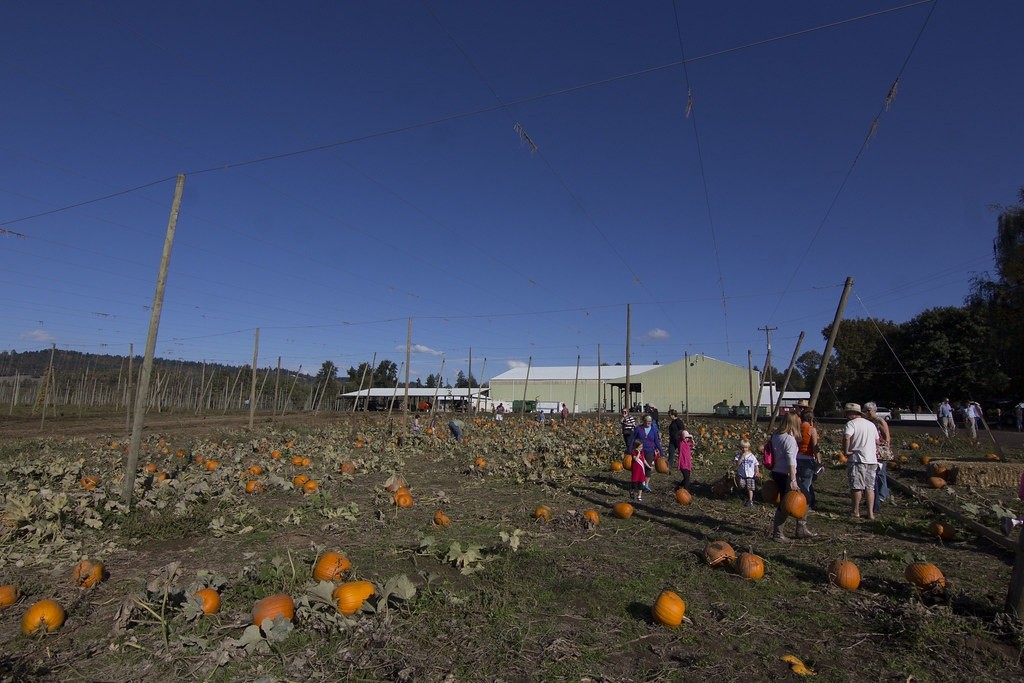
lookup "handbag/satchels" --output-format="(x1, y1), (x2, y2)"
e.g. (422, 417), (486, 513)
(763, 439), (773, 469)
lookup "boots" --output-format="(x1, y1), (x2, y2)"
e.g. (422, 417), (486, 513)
(796, 513), (818, 538)
(773, 510), (792, 544)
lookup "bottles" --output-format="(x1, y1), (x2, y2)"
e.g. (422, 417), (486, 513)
(815, 466), (824, 475)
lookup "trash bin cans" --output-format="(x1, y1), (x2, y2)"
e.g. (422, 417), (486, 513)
(733, 406), (749, 417)
(715, 406), (729, 415)
(513, 400), (538, 413)
(753, 406), (767, 417)
(780, 407), (794, 415)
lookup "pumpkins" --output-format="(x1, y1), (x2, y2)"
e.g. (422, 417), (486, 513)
(0, 417), (1001, 636)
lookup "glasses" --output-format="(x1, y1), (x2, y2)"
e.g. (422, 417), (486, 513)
(864, 409), (872, 413)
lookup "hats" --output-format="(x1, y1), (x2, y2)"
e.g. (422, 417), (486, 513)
(840, 402), (868, 417)
(792, 399), (812, 411)
(677, 429), (692, 439)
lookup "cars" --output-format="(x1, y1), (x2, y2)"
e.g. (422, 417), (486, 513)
(357, 398), (404, 412)
(876, 406), (893, 421)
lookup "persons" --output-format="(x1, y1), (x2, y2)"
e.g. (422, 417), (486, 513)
(994, 408), (1002, 431)
(535, 411), (545, 421)
(862, 402), (894, 513)
(770, 412), (818, 543)
(413, 415), (420, 434)
(620, 407), (636, 445)
(734, 439), (759, 506)
(629, 439), (654, 501)
(676, 430), (695, 489)
(496, 403), (504, 423)
(550, 409), (554, 419)
(561, 404), (569, 424)
(842, 403), (880, 519)
(796, 409), (821, 504)
(648, 403), (658, 424)
(628, 414), (664, 491)
(939, 398), (956, 435)
(447, 419), (461, 441)
(963, 400), (982, 438)
(667, 409), (685, 469)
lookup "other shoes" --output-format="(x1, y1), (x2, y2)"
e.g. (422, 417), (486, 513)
(807, 509), (816, 513)
(1001, 516), (1013, 536)
(851, 512), (860, 518)
(629, 489), (635, 499)
(642, 480), (652, 492)
(637, 495), (642, 501)
(745, 500), (753, 507)
(868, 513), (875, 519)
